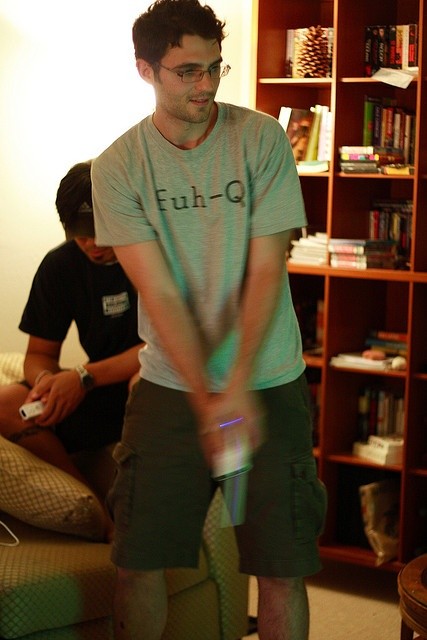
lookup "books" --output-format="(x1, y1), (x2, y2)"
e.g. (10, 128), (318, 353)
(347, 387), (403, 462)
(328, 201), (415, 269)
(365, 23), (419, 81)
(278, 104), (331, 174)
(284, 30), (293, 78)
(331, 331), (408, 372)
(286, 231), (327, 267)
(315, 300), (325, 357)
(335, 94), (414, 173)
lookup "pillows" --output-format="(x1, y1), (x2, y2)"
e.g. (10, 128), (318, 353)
(0, 436), (111, 541)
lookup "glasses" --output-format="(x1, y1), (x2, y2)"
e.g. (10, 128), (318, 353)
(149, 57), (232, 84)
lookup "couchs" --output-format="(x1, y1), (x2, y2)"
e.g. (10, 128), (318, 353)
(0, 351), (247, 640)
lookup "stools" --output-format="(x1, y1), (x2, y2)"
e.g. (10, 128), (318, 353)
(397, 556), (427, 640)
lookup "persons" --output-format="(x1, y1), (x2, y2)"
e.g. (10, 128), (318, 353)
(91, 0), (327, 639)
(0, 160), (146, 504)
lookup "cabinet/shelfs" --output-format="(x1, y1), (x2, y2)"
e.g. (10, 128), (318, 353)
(254, 1), (427, 604)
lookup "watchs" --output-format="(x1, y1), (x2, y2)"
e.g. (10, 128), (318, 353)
(75, 365), (96, 391)
